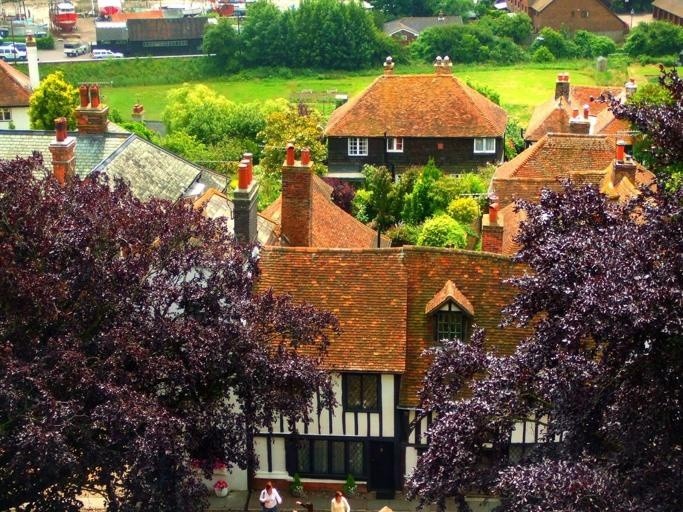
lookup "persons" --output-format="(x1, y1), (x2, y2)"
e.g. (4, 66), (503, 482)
(258, 481), (284, 512)
(330, 489), (350, 512)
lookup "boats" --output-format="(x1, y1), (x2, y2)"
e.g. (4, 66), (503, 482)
(48, 1), (77, 33)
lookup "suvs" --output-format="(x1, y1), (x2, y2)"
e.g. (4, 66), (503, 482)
(0, 42), (26, 61)
(63, 41), (85, 57)
(92, 49), (123, 59)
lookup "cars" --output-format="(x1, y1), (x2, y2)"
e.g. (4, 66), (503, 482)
(95, 16), (111, 23)
